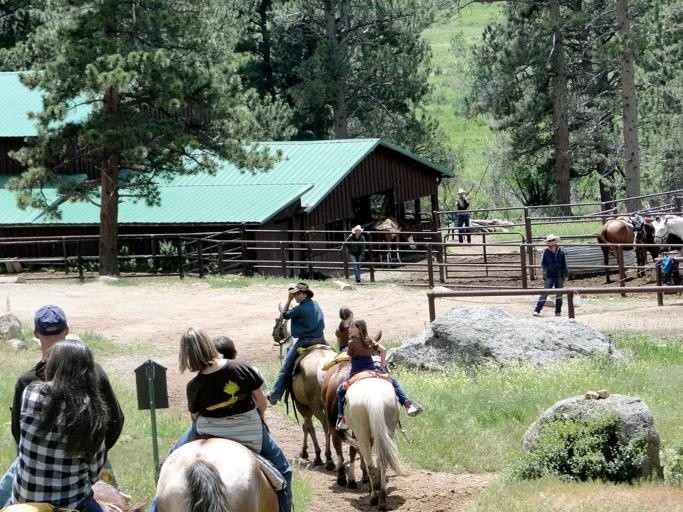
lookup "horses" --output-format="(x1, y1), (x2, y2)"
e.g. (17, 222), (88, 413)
(652, 213), (683, 247)
(154, 436), (281, 512)
(271, 300), (340, 472)
(341, 351), (410, 512)
(596, 216), (662, 284)
(361, 215), (417, 269)
(319, 327), (383, 489)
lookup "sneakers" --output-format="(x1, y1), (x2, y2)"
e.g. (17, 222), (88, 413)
(335, 420), (349, 430)
(406, 407), (423, 416)
(265, 391), (277, 405)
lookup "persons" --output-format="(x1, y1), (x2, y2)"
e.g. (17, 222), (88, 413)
(629, 216), (648, 278)
(375, 216), (403, 258)
(531, 232), (567, 319)
(650, 215), (668, 253)
(343, 225), (367, 283)
(266, 278), (329, 407)
(454, 188), (471, 244)
(8, 303), (124, 512)
(158, 324), (295, 509)
(656, 251), (675, 285)
(331, 319), (424, 434)
(210, 335), (239, 360)
(337, 303), (383, 352)
(0, 339), (109, 512)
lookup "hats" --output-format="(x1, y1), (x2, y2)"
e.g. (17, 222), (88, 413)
(543, 234), (560, 242)
(351, 224), (364, 234)
(33, 304), (66, 336)
(456, 188), (467, 194)
(287, 283), (313, 298)
